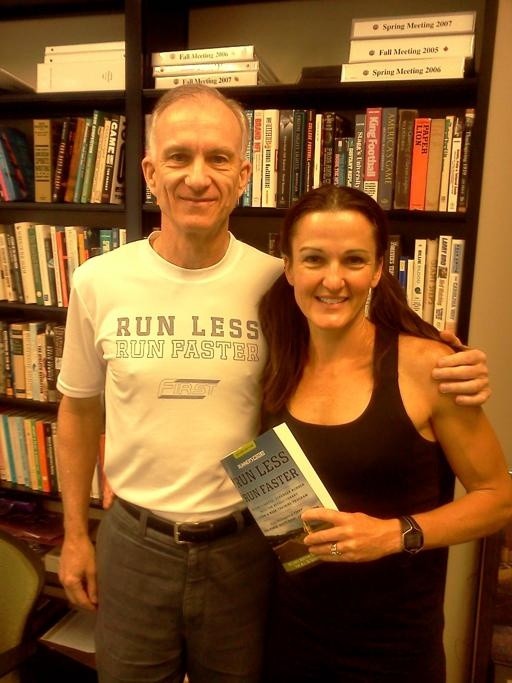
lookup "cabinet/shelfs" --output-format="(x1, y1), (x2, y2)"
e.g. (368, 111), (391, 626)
(0, 0), (499, 683)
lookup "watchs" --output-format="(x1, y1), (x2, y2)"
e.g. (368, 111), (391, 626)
(400, 513), (424, 554)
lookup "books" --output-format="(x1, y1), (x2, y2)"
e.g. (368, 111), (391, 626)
(238, 108), (483, 212)
(1, 222), (126, 307)
(1, 321), (65, 403)
(39, 611), (97, 668)
(219, 421), (339, 576)
(2, 522), (95, 598)
(341, 10), (485, 83)
(1, 111), (128, 205)
(152, 46), (282, 89)
(36, 41), (126, 92)
(1, 407), (107, 498)
(267, 231), (474, 340)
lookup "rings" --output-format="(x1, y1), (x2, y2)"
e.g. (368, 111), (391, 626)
(331, 543), (342, 557)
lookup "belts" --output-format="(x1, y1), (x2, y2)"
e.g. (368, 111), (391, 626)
(119, 499), (254, 542)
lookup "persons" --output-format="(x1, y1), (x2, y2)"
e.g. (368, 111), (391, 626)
(54, 86), (492, 677)
(267, 185), (512, 677)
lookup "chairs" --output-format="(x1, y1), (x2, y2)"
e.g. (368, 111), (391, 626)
(0, 528), (47, 683)
